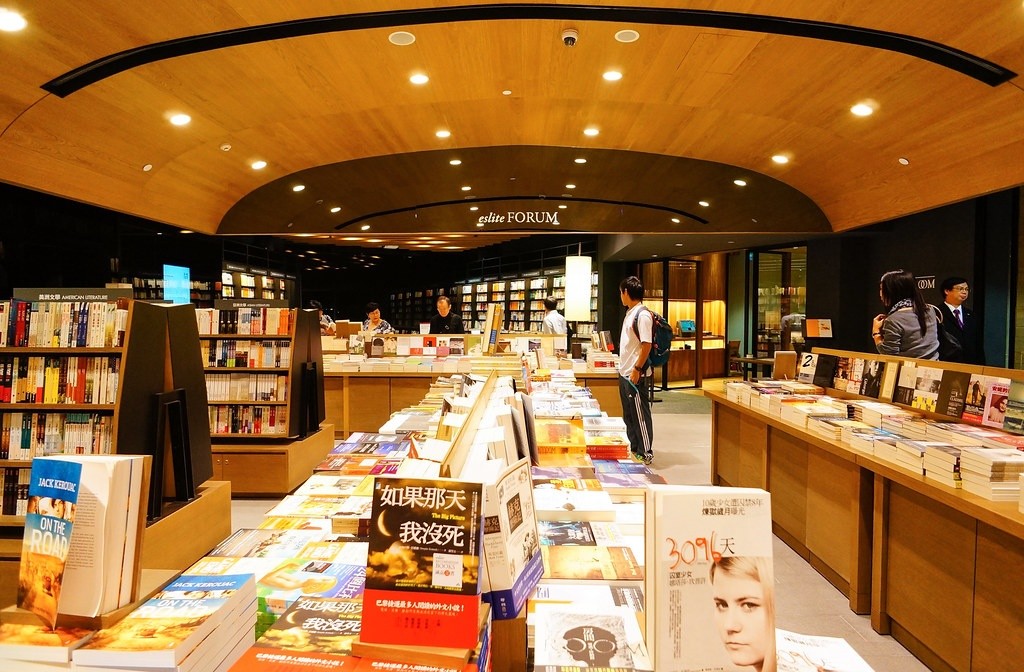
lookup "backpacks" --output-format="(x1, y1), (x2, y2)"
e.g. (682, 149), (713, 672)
(632, 306), (673, 372)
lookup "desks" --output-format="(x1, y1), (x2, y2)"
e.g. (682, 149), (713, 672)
(855, 366), (1024, 672)
(732, 358), (774, 381)
(703, 346), (985, 614)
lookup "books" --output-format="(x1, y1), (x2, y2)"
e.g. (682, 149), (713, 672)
(0, 272), (1024, 672)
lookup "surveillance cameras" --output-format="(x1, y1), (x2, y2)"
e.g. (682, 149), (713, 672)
(562, 28), (579, 48)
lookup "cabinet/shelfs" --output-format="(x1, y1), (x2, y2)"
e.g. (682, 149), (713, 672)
(390, 271), (599, 339)
(0, 269), (334, 570)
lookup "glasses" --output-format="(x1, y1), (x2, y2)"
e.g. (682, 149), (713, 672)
(947, 287), (970, 292)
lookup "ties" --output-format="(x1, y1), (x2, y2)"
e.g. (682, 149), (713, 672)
(953, 309), (963, 331)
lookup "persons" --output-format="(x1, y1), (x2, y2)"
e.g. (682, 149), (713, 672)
(541, 296), (567, 334)
(972, 381), (981, 404)
(560, 308), (574, 353)
(872, 269), (940, 361)
(781, 303), (806, 338)
(935, 277), (986, 366)
(26, 496), (41, 514)
(709, 556), (777, 672)
(363, 302), (391, 334)
(51, 498), (66, 519)
(980, 393), (986, 407)
(430, 296), (466, 334)
(67, 503), (76, 523)
(859, 360), (885, 399)
(618, 276), (655, 465)
(311, 302), (336, 336)
(988, 396), (1008, 423)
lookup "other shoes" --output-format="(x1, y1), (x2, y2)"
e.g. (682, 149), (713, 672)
(633, 452), (653, 465)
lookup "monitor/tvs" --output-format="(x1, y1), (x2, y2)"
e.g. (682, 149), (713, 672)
(677, 319), (696, 337)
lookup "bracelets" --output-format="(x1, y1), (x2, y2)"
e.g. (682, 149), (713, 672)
(872, 332), (881, 338)
(635, 366), (642, 371)
